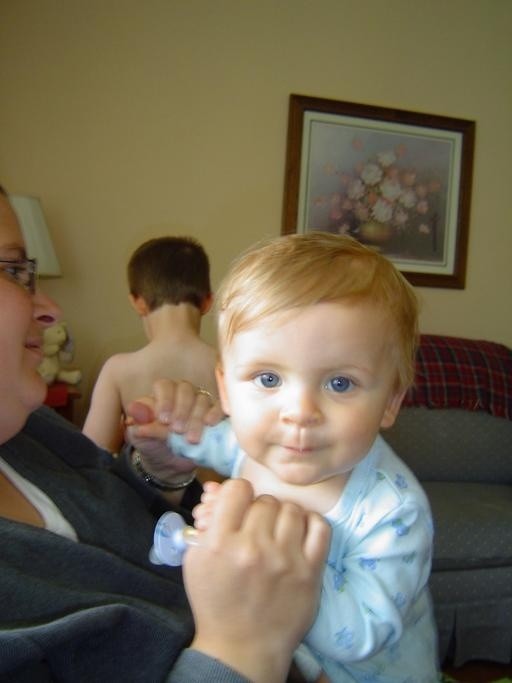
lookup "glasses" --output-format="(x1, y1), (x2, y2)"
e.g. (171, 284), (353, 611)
(0, 255), (37, 296)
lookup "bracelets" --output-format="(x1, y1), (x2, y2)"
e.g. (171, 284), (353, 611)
(135, 449), (197, 492)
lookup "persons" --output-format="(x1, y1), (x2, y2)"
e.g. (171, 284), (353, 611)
(82, 237), (220, 455)
(124, 232), (437, 683)
(0, 182), (333, 683)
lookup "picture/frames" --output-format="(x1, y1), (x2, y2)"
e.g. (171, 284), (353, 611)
(280, 94), (477, 292)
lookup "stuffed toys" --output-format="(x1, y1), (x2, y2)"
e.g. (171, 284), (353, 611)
(37, 324), (81, 385)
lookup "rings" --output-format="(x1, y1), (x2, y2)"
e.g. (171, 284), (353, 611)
(195, 387), (215, 407)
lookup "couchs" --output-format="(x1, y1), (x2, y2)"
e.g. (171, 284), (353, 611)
(378, 335), (510, 668)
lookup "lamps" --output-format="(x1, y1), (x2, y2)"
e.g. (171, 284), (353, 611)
(11, 196), (64, 279)
